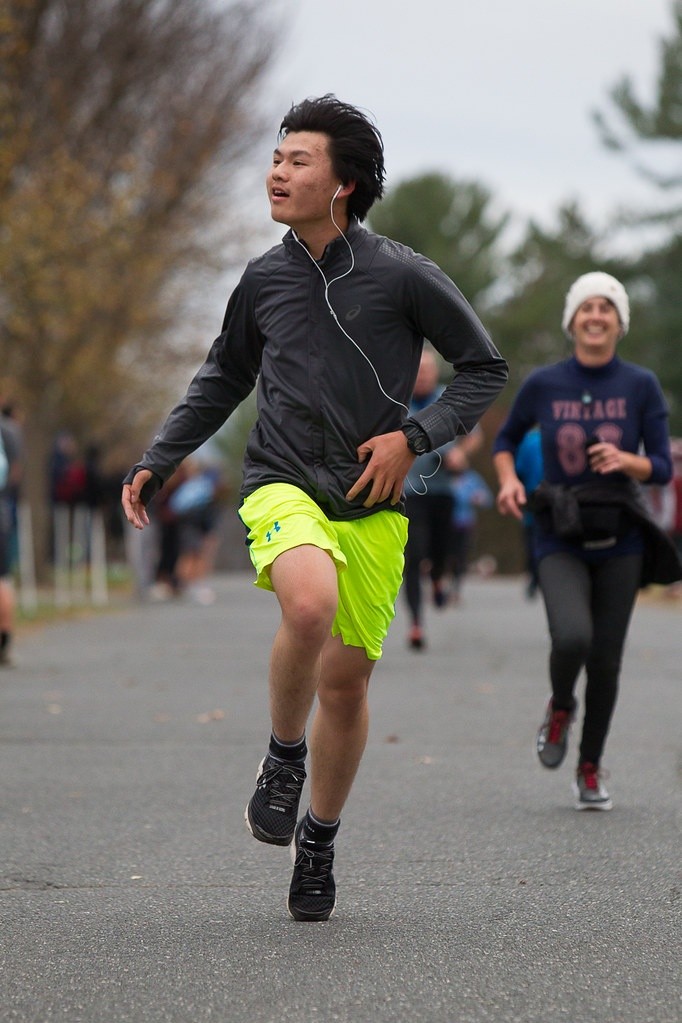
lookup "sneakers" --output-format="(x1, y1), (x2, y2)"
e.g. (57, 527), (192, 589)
(535, 697), (577, 769)
(287, 820), (335, 924)
(245, 754), (307, 845)
(570, 769), (616, 811)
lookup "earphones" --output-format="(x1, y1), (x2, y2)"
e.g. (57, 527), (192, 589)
(334, 184), (345, 199)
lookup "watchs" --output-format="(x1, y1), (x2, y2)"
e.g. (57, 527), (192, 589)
(401, 422), (429, 456)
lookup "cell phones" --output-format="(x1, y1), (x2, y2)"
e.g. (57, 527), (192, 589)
(584, 435), (606, 463)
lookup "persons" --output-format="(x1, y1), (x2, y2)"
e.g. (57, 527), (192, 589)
(445, 446), (491, 603)
(493, 271), (675, 814)
(404, 356), (456, 653)
(0, 389), (29, 667)
(42, 405), (233, 605)
(125, 91), (509, 921)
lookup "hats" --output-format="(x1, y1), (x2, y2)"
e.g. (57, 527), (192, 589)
(562, 271), (633, 340)
